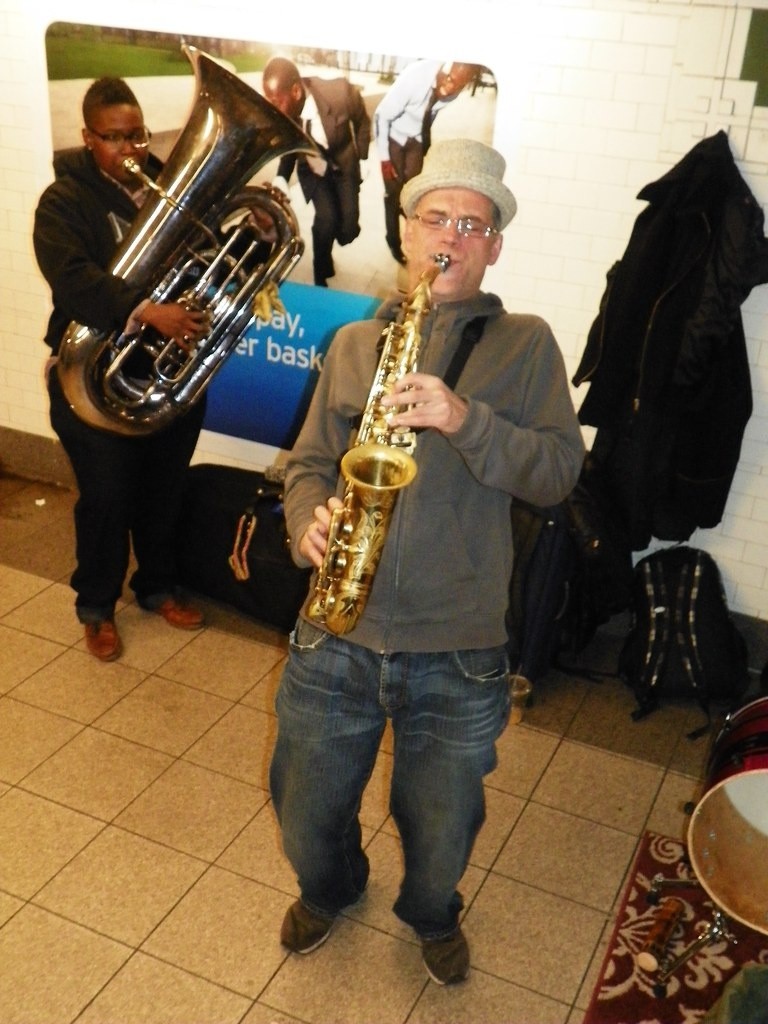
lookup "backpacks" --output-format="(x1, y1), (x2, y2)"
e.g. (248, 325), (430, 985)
(618, 546), (752, 740)
(505, 451), (632, 709)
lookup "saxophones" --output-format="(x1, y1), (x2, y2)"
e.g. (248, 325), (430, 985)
(300, 251), (451, 636)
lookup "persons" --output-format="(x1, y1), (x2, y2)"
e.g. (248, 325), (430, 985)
(374, 61), (483, 266)
(31, 73), (289, 660)
(268, 136), (585, 987)
(261, 57), (373, 286)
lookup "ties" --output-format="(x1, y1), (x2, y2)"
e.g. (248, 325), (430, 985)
(422, 95), (439, 156)
(306, 119), (333, 169)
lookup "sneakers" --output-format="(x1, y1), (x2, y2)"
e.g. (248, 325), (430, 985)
(280, 899), (335, 954)
(421, 927), (470, 985)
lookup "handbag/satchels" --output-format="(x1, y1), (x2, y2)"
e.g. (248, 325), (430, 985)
(178, 464), (315, 631)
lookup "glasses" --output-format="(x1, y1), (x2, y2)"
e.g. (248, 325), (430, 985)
(86, 125), (151, 150)
(412, 214), (497, 238)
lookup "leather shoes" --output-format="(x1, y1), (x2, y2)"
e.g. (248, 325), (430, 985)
(392, 245), (407, 264)
(85, 617), (122, 662)
(154, 593), (204, 629)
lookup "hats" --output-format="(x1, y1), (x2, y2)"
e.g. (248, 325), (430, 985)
(400, 139), (517, 233)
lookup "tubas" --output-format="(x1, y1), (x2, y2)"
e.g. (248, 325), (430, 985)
(53, 38), (334, 438)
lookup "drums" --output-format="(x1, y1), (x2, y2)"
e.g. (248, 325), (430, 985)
(683, 695), (768, 939)
